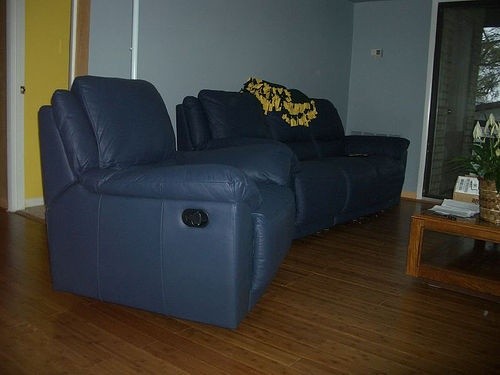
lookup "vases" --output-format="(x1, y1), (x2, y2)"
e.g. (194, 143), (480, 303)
(477, 178), (500, 225)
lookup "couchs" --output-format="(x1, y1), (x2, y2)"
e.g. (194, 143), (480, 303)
(175, 76), (411, 240)
(38, 75), (298, 331)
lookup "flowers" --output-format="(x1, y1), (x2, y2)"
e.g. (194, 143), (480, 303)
(447, 110), (500, 195)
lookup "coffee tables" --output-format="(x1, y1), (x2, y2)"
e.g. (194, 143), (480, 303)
(404, 205), (500, 298)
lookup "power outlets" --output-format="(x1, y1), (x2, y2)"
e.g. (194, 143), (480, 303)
(376, 133), (387, 136)
(351, 131), (361, 135)
(389, 134), (400, 137)
(364, 131), (374, 136)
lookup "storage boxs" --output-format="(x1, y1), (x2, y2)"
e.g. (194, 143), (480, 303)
(452, 173), (479, 204)
